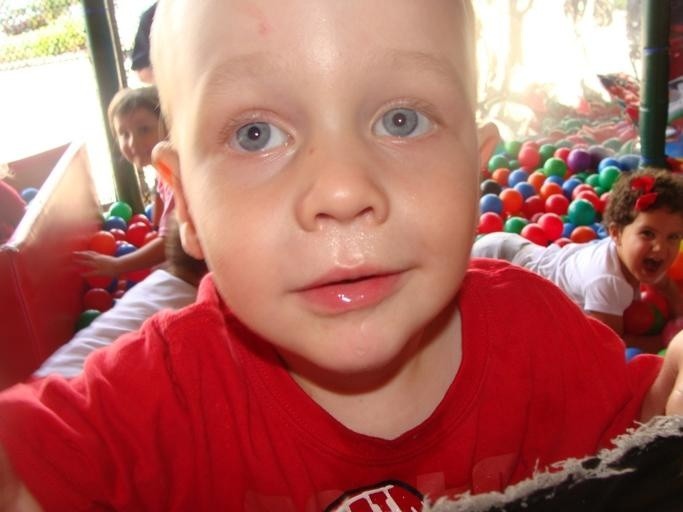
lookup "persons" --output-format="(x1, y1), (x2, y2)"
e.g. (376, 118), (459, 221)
(24, 206), (208, 376)
(0, 0), (682, 511)
(467, 168), (681, 349)
(61, 87), (171, 284)
